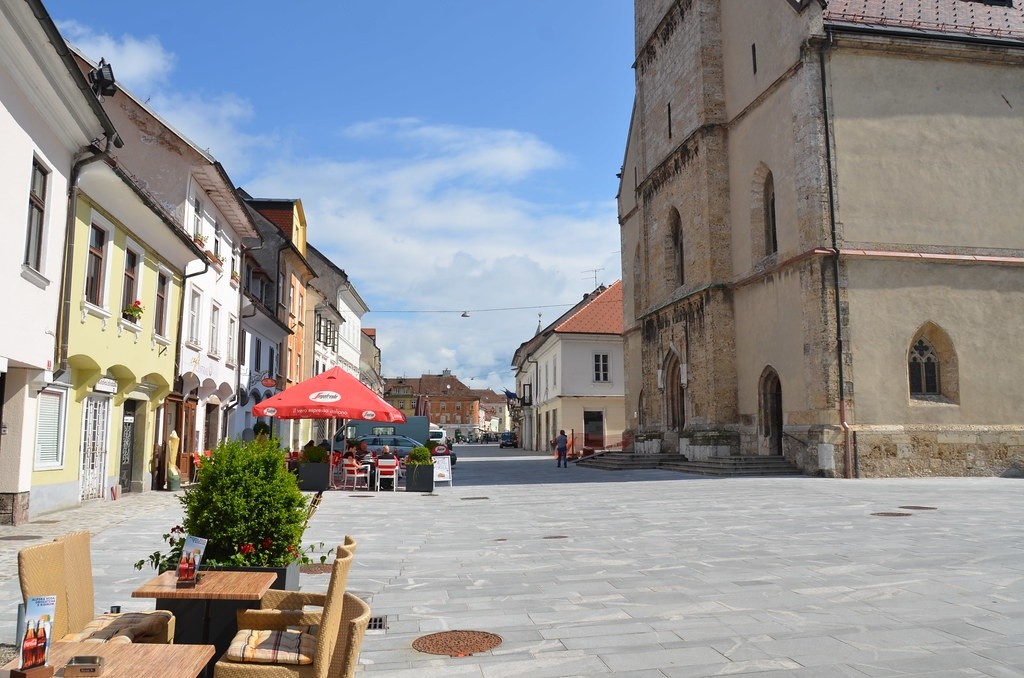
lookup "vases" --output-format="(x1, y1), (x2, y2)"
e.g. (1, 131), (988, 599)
(154, 555), (302, 674)
(122, 312), (137, 324)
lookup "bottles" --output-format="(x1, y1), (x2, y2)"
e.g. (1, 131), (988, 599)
(178, 549), (200, 580)
(22, 615), (52, 670)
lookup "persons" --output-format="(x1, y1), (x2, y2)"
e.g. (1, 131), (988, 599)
(556, 429), (567, 468)
(304, 438), (331, 452)
(344, 441), (399, 490)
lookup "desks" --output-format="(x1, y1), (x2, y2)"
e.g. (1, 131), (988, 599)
(129, 566), (278, 678)
(0, 641), (217, 678)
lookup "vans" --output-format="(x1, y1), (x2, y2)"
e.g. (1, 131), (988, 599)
(325, 415), (431, 458)
(499, 431), (518, 448)
(428, 430), (447, 448)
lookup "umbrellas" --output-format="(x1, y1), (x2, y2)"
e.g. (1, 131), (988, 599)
(252, 366), (407, 484)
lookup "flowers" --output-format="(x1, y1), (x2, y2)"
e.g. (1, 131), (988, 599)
(121, 300), (144, 320)
(128, 429), (335, 568)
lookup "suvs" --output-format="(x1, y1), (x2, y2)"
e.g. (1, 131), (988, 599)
(352, 434), (457, 474)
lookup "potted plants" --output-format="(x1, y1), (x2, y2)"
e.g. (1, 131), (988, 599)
(401, 446), (437, 493)
(296, 444), (331, 491)
(194, 232), (226, 265)
(232, 271), (242, 283)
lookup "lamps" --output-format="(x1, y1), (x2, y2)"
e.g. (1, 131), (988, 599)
(88, 56), (118, 103)
(249, 369), (278, 390)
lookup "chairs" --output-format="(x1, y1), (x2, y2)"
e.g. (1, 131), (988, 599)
(213, 536), (370, 678)
(17, 529), (175, 649)
(341, 457), (399, 493)
